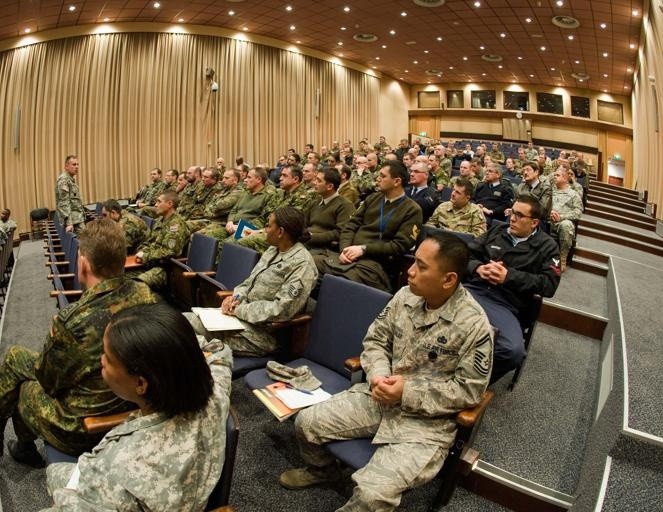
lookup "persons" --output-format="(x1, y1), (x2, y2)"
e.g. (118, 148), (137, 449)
(1, 216), (156, 468)
(454, 197), (564, 389)
(1, 207), (15, 251)
(195, 144), (327, 260)
(392, 131), (456, 220)
(457, 137), (513, 224)
(128, 157), (223, 229)
(123, 191), (190, 285)
(100, 201), (149, 255)
(424, 179), (487, 238)
(327, 136), (391, 200)
(280, 233), (494, 511)
(516, 140), (589, 272)
(311, 161), (424, 285)
(38, 302), (235, 511)
(179, 208), (319, 349)
(55, 156), (86, 235)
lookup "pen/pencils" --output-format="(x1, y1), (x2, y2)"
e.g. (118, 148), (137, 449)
(229, 294), (239, 312)
(285, 384), (313, 395)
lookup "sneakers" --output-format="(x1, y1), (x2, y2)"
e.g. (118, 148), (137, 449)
(7, 440), (43, 468)
(560, 255), (566, 272)
(280, 463), (344, 489)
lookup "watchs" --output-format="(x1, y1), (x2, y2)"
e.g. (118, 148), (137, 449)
(361, 243), (367, 252)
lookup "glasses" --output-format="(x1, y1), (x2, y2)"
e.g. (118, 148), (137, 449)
(510, 210), (535, 219)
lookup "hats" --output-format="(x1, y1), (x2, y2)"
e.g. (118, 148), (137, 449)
(265, 360), (322, 391)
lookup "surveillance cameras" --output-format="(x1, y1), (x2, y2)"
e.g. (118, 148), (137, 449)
(211, 82), (219, 92)
